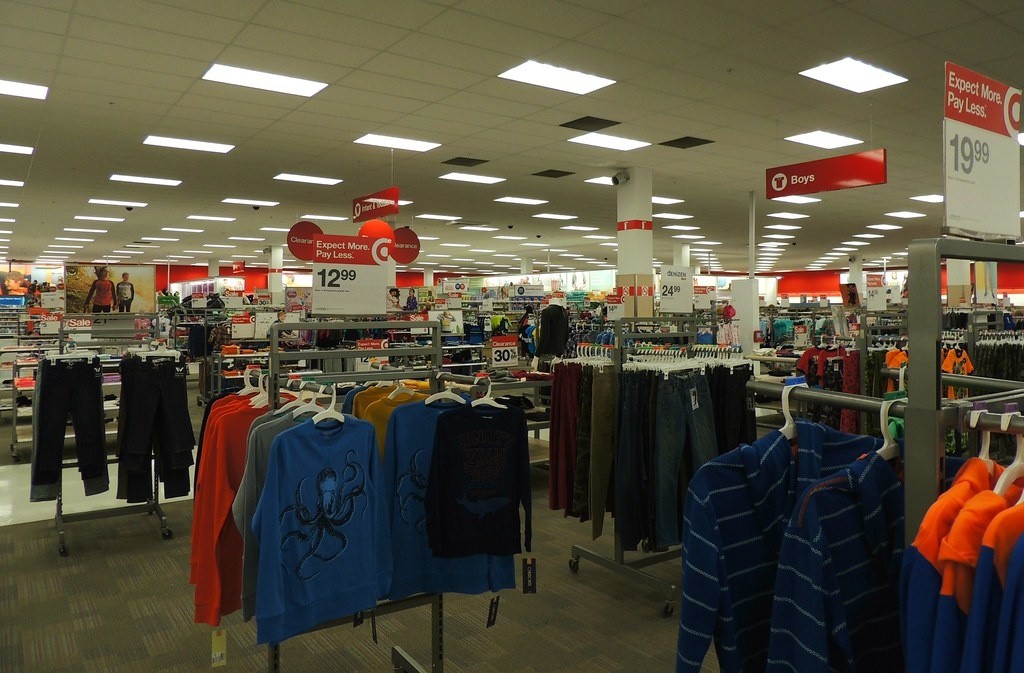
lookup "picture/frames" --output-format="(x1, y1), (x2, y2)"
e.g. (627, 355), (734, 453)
(64, 264), (155, 314)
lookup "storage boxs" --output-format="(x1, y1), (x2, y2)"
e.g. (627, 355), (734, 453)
(492, 335), (519, 368)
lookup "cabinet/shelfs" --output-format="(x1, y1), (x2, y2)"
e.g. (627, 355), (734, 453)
(567, 300), (587, 317)
(175, 311), (265, 355)
(209, 352), (269, 395)
(442, 345), (487, 368)
(462, 301), (540, 321)
(0, 310), (41, 337)
(0, 345), (102, 410)
(11, 355), (121, 440)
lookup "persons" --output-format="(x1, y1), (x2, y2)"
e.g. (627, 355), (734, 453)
(722, 300), (737, 322)
(386, 287), (403, 310)
(402, 289), (418, 311)
(3, 270), (29, 295)
(114, 272), (134, 313)
(846, 284), (859, 308)
(517, 305), (533, 328)
(531, 298), (570, 405)
(83, 266), (118, 313)
(265, 311), (297, 339)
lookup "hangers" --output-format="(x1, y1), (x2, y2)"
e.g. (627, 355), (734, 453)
(470, 377), (506, 412)
(129, 339), (182, 365)
(44, 343), (94, 366)
(993, 412), (1024, 496)
(238, 366), (344, 425)
(554, 343), (752, 377)
(774, 379), (810, 440)
(424, 372), (465, 405)
(697, 319), (741, 335)
(942, 306), (1013, 315)
(873, 394), (908, 459)
(818, 330), (1024, 353)
(967, 407), (993, 477)
(360, 359), (416, 400)
(574, 322), (661, 335)
